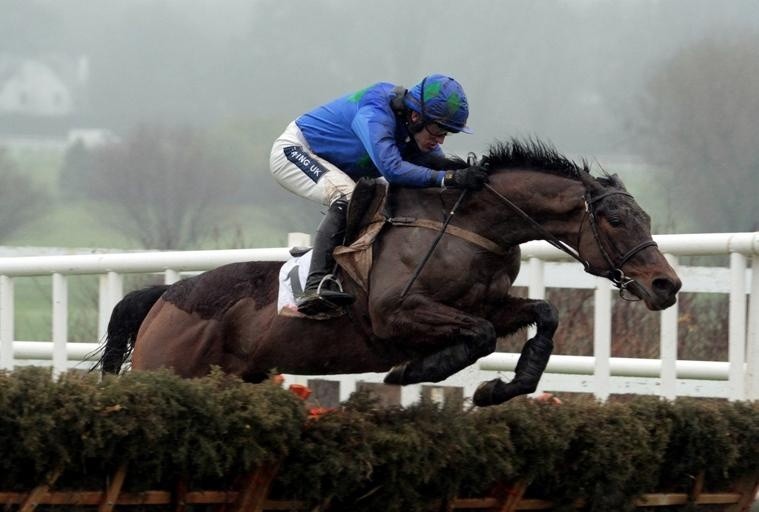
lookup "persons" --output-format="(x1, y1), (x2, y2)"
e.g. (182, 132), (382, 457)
(268, 73), (490, 316)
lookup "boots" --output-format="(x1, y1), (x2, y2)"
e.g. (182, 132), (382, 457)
(298, 200), (356, 316)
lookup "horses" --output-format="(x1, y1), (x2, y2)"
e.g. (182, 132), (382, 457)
(74, 132), (683, 408)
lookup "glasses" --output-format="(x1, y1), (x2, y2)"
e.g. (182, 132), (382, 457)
(421, 77), (449, 136)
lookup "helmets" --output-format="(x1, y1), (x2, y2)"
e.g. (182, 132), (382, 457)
(403, 74), (472, 134)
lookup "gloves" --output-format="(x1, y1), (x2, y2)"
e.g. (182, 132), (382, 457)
(445, 166), (488, 189)
